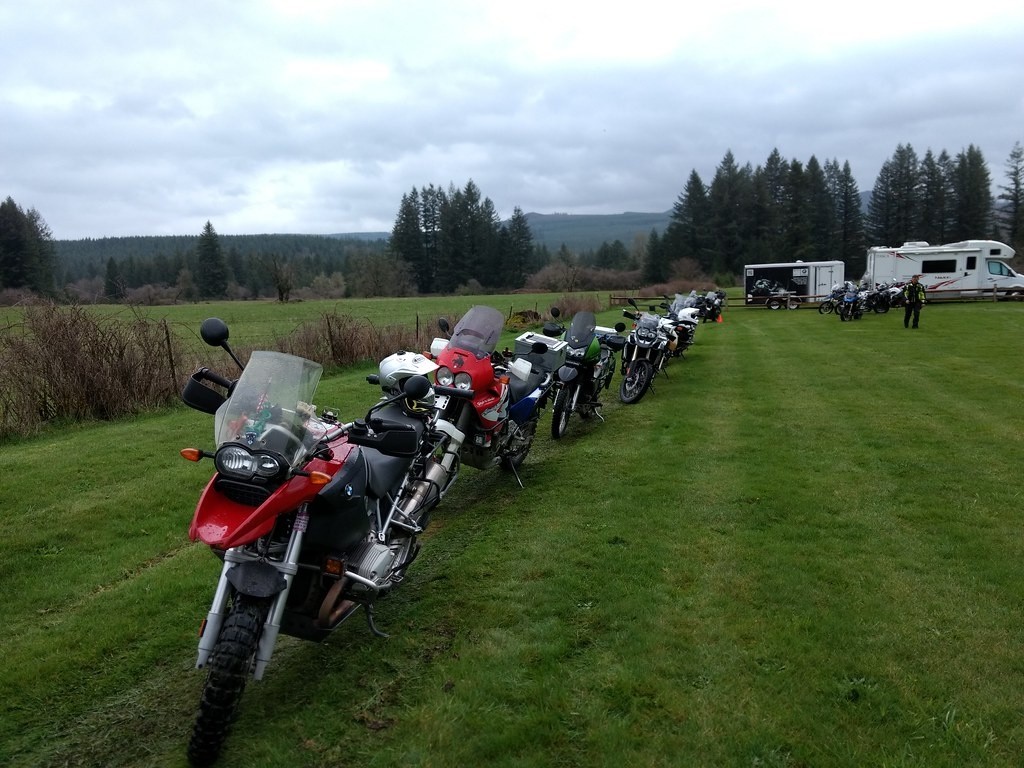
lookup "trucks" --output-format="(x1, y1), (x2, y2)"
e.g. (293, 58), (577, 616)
(865, 239), (1024, 302)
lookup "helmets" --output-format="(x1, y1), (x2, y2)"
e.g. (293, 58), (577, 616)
(379, 350), (440, 407)
(678, 308), (700, 324)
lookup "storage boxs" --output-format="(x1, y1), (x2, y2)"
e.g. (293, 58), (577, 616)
(593, 326), (618, 350)
(678, 308), (700, 323)
(717, 291), (726, 299)
(515, 331), (569, 372)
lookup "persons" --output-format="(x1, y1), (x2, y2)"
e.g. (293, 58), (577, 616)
(904, 274), (925, 328)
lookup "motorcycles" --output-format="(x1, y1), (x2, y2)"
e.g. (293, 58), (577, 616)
(180, 314), (450, 768)
(543, 306), (626, 440)
(818, 271), (909, 322)
(622, 288), (730, 405)
(418, 304), (567, 534)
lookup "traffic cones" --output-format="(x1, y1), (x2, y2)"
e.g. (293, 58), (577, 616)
(717, 315), (723, 323)
